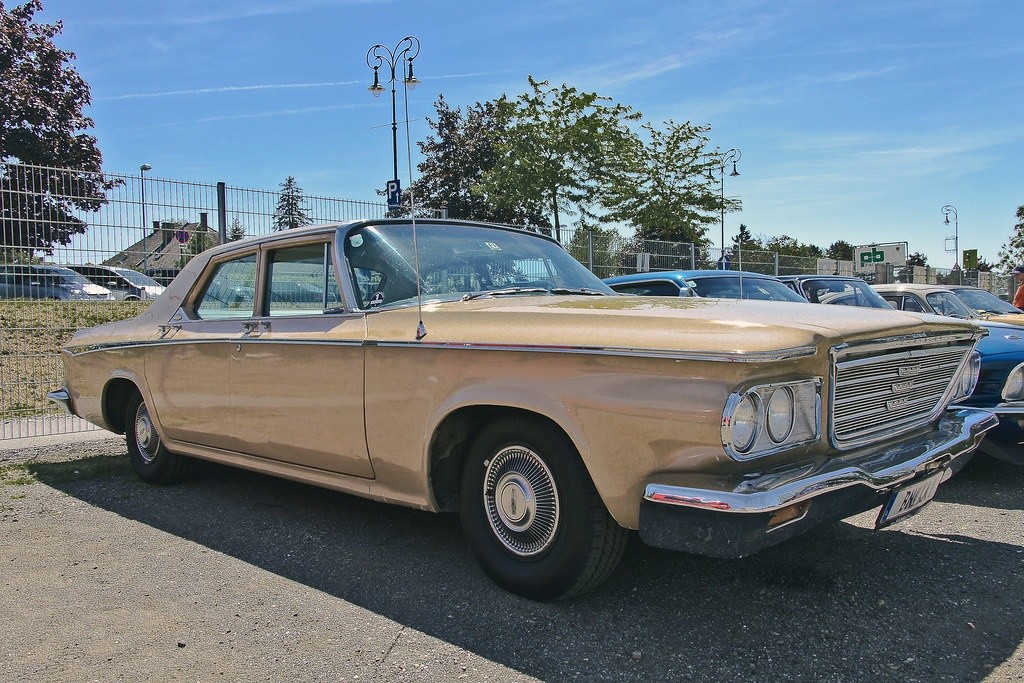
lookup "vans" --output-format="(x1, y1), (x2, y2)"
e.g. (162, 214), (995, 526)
(0, 264), (116, 300)
(55, 265), (168, 301)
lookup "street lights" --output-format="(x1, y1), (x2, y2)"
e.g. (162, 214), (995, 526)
(941, 205), (959, 263)
(366, 35), (421, 179)
(140, 163), (152, 273)
(703, 147), (742, 256)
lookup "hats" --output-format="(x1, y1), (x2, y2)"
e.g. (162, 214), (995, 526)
(1010, 266), (1024, 274)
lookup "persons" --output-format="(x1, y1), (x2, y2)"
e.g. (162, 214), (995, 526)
(1010, 266), (1024, 311)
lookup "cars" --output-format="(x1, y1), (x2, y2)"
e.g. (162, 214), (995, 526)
(818, 283), (990, 321)
(940, 284), (1024, 326)
(600, 269), (1024, 453)
(45, 219), (1004, 605)
(774, 274), (896, 310)
